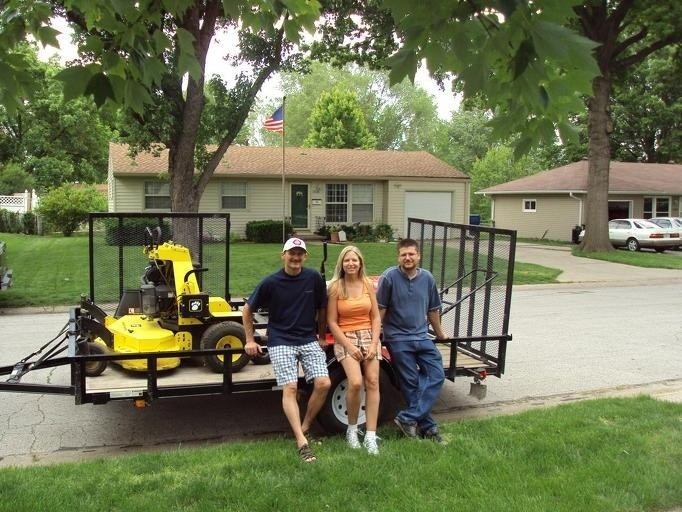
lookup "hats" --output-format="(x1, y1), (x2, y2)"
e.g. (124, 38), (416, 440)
(282, 238), (308, 254)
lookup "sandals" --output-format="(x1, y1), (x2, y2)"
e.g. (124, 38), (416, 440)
(298, 444), (316, 462)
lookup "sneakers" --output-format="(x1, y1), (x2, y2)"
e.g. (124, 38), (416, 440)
(394, 417), (417, 439)
(347, 430), (362, 449)
(363, 435), (379, 455)
(424, 427), (442, 444)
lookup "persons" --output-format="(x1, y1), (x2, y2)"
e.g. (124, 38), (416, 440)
(327, 245), (382, 455)
(242, 238), (332, 463)
(373, 239), (449, 445)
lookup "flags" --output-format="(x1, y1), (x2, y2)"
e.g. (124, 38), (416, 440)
(264, 105), (283, 134)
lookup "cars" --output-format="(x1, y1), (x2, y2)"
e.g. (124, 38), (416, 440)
(577, 216), (682, 253)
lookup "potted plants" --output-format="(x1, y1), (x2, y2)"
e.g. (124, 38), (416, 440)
(328, 226), (342, 242)
